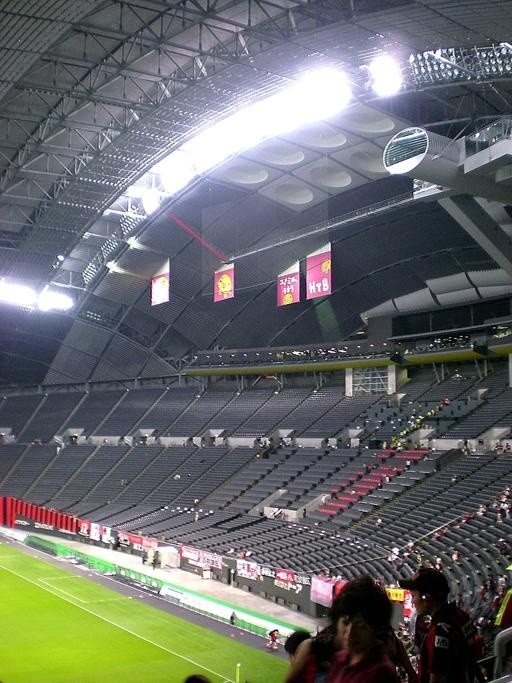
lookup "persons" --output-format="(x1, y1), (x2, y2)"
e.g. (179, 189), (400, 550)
(283, 394), (512, 683)
(230, 611), (237, 626)
(141, 548), (149, 565)
(266, 626), (279, 650)
(152, 550), (159, 569)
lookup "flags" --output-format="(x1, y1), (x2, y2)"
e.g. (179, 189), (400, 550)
(213, 262), (235, 303)
(305, 242), (332, 300)
(149, 255), (171, 307)
(275, 259), (301, 307)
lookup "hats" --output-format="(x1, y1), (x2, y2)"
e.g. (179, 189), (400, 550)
(398, 567), (451, 601)
